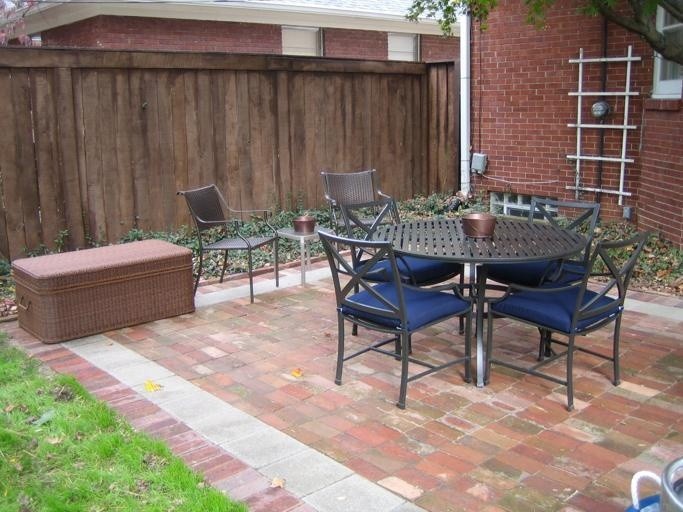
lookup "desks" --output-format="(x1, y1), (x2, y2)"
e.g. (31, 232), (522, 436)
(373, 218), (586, 386)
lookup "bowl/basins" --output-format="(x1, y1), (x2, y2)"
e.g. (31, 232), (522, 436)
(291, 215), (317, 233)
(462, 213), (497, 240)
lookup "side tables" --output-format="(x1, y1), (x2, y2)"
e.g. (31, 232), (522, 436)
(276, 226), (337, 285)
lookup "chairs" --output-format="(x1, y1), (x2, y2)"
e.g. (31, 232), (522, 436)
(318, 231), (472, 409)
(476, 199), (602, 357)
(339, 198), (466, 358)
(485, 234), (648, 410)
(321, 170), (392, 270)
(177, 184), (279, 304)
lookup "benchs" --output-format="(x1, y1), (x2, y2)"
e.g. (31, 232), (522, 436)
(11, 239), (195, 344)
